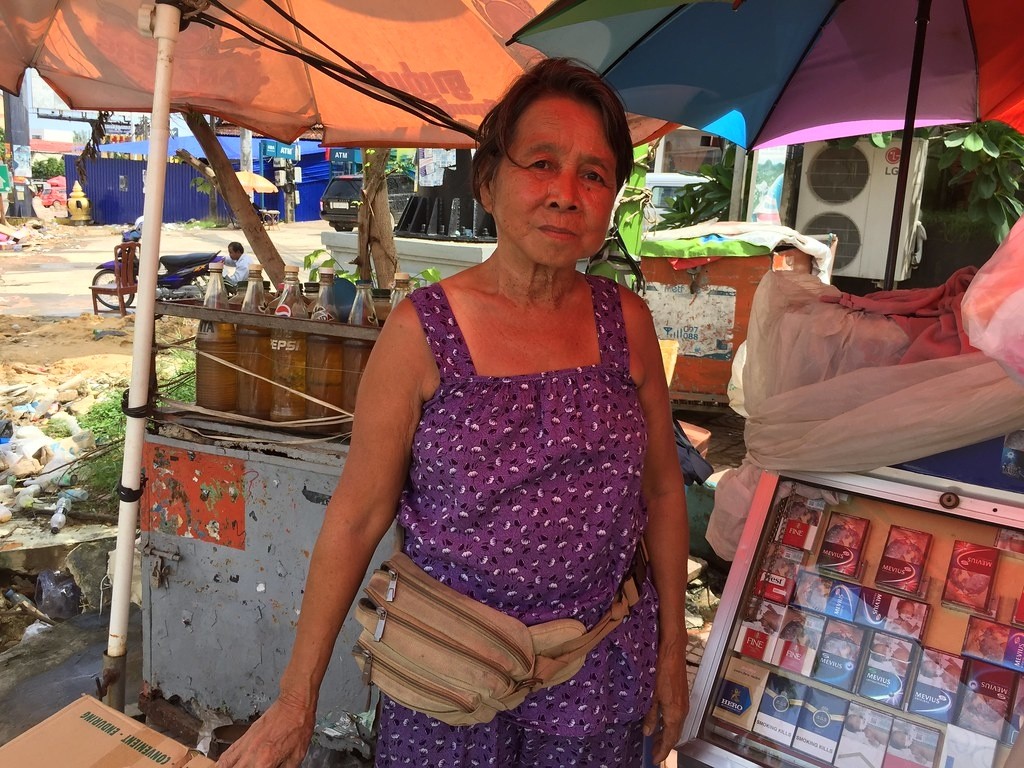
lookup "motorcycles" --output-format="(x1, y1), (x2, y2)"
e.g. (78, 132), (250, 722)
(92, 224), (237, 310)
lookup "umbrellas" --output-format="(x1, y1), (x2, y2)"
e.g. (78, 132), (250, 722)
(235, 170), (279, 193)
(506, 0), (1024, 294)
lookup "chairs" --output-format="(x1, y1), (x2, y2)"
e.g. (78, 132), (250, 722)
(223, 202), (238, 229)
(89, 241), (141, 317)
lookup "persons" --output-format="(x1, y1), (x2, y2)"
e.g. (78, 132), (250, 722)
(213, 55), (690, 767)
(222, 242), (254, 285)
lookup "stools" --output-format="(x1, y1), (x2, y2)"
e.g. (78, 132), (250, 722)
(266, 210), (281, 231)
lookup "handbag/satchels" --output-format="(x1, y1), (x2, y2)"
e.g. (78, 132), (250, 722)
(351, 524), (651, 727)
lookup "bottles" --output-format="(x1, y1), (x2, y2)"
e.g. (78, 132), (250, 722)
(391, 273), (411, 315)
(0, 503), (12, 523)
(307, 267), (342, 432)
(268, 265), (307, 419)
(58, 490), (88, 502)
(227, 280), (320, 318)
(5, 589), (36, 607)
(20, 481), (51, 497)
(372, 288), (391, 327)
(32, 388), (59, 422)
(49, 497), (72, 534)
(235, 264), (270, 419)
(342, 280), (379, 433)
(196, 263), (235, 410)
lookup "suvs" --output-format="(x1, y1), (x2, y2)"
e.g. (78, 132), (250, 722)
(319, 172), (414, 233)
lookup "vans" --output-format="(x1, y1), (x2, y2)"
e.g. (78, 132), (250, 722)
(642, 173), (720, 237)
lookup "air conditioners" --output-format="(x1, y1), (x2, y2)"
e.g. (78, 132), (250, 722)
(795, 136), (929, 281)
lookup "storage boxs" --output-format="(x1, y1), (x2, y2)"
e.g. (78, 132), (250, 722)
(712, 493), (1024, 768)
(0, 694), (214, 768)
(640, 238), (837, 396)
